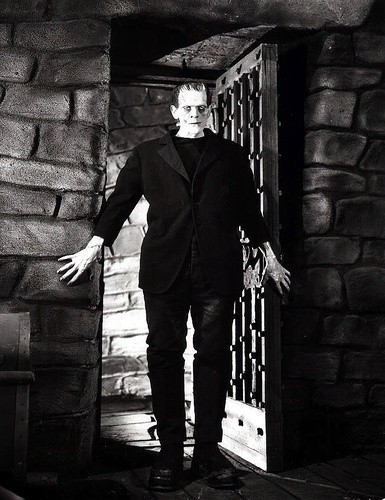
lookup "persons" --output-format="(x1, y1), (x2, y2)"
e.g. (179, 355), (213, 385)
(57, 80), (292, 489)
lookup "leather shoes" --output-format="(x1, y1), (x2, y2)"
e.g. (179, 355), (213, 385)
(147, 446), (184, 491)
(190, 443), (237, 486)
